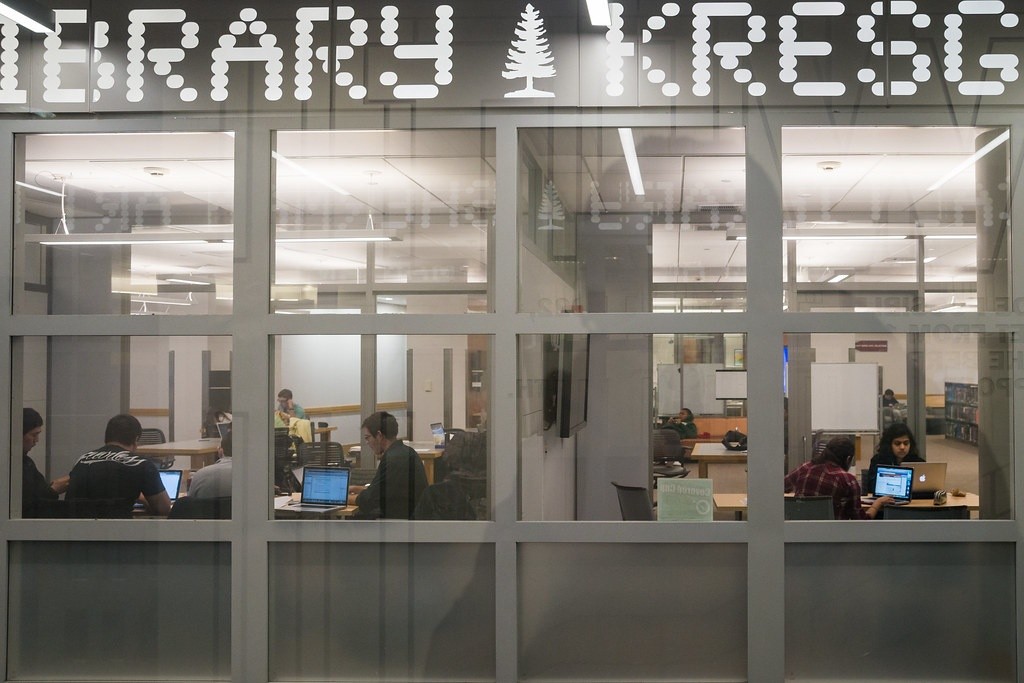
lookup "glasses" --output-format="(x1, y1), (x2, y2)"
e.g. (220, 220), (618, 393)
(680, 412), (688, 416)
(277, 399), (288, 403)
(363, 434), (373, 440)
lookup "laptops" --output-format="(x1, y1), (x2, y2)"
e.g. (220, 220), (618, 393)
(133, 469), (184, 508)
(900, 462), (947, 498)
(859, 464), (914, 505)
(273, 466), (350, 514)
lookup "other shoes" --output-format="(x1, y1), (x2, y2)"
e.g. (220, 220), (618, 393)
(673, 460), (681, 466)
(652, 459), (668, 465)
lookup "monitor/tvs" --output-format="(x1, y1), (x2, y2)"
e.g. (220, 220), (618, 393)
(555, 310), (590, 438)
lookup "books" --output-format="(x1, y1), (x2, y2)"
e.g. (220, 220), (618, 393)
(945, 385), (979, 443)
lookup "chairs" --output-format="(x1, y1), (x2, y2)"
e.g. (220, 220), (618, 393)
(612, 429), (972, 519)
(137, 422), (489, 519)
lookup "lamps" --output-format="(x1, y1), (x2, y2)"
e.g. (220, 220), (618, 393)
(14, 161), (402, 316)
(727, 221), (980, 284)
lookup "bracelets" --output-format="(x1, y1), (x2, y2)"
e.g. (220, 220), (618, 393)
(871, 506), (878, 512)
(288, 408), (294, 410)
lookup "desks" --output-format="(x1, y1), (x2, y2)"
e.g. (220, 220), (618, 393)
(130, 492), (359, 520)
(711, 492), (981, 519)
(692, 441), (747, 495)
(135, 437), (222, 467)
(356, 446), (444, 484)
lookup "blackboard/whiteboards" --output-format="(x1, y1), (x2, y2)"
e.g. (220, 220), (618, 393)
(657, 363), (725, 417)
(811, 362), (879, 432)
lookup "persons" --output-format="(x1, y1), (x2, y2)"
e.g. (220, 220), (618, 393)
(865, 423), (925, 496)
(656, 408), (697, 439)
(22, 408), (70, 518)
(186, 431), (232, 498)
(882, 389), (899, 407)
(275, 389), (306, 429)
(347, 411), (429, 520)
(56, 414), (171, 519)
(784, 437), (895, 520)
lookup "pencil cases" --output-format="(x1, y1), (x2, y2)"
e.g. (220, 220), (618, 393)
(934, 490), (946, 504)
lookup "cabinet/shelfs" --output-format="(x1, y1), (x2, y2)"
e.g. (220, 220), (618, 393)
(944, 381), (980, 447)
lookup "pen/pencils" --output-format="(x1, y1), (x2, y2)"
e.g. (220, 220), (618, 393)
(742, 451), (747, 453)
(198, 440), (210, 442)
